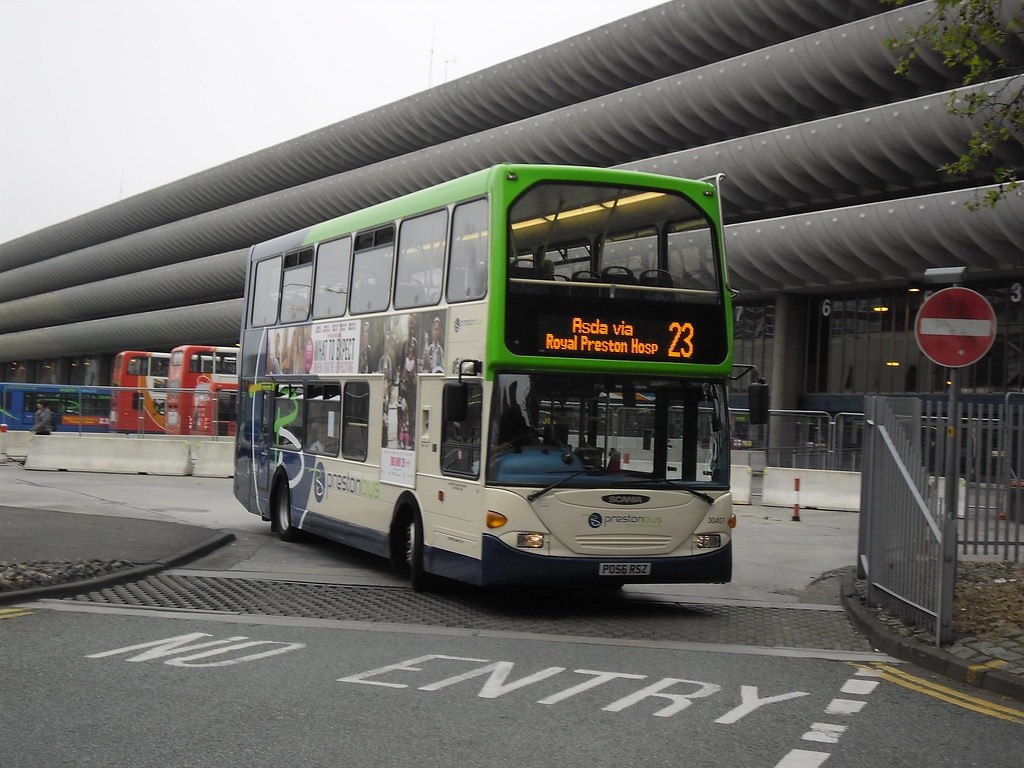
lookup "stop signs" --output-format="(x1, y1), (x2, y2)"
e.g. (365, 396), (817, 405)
(913, 287), (999, 369)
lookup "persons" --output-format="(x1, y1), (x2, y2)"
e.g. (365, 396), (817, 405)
(309, 428), (329, 452)
(542, 258), (555, 280)
(488, 404), (542, 456)
(360, 314), (444, 450)
(28, 399), (51, 435)
(266, 324), (312, 375)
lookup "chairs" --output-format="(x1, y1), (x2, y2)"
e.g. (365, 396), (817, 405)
(260, 258), (677, 325)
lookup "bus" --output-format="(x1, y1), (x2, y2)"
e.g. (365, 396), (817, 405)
(187, 375), (239, 436)
(107, 351), (237, 433)
(231, 161), (773, 596)
(163, 344), (239, 436)
(0, 383), (144, 433)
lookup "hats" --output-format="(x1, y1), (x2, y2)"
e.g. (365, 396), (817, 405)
(362, 321), (369, 332)
(433, 317), (440, 322)
(408, 337), (417, 347)
(36, 399), (48, 407)
